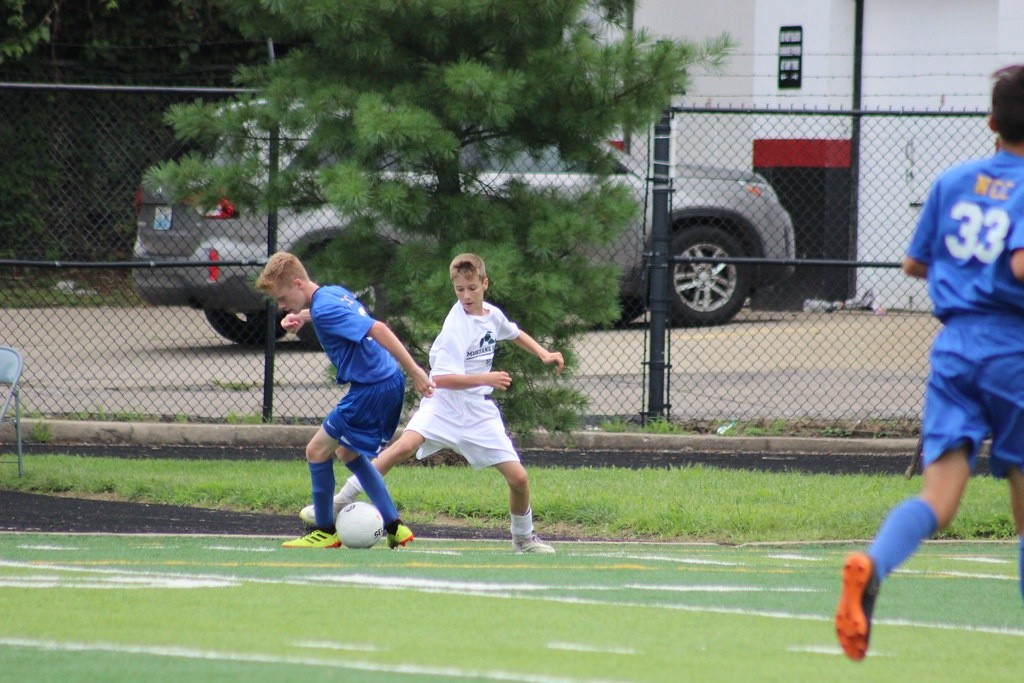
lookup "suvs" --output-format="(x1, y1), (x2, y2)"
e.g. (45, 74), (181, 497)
(130, 95), (798, 354)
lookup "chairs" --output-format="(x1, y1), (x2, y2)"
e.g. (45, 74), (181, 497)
(0, 344), (24, 479)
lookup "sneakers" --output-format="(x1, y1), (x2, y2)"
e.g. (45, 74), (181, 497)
(299, 498), (346, 526)
(283, 528), (342, 549)
(387, 524), (414, 548)
(836, 551), (881, 659)
(513, 536), (556, 555)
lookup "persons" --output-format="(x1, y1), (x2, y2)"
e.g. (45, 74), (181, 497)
(834, 64), (1024, 661)
(300, 253), (564, 553)
(256, 252), (437, 549)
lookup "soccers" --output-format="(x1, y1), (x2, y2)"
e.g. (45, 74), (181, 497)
(334, 501), (384, 549)
